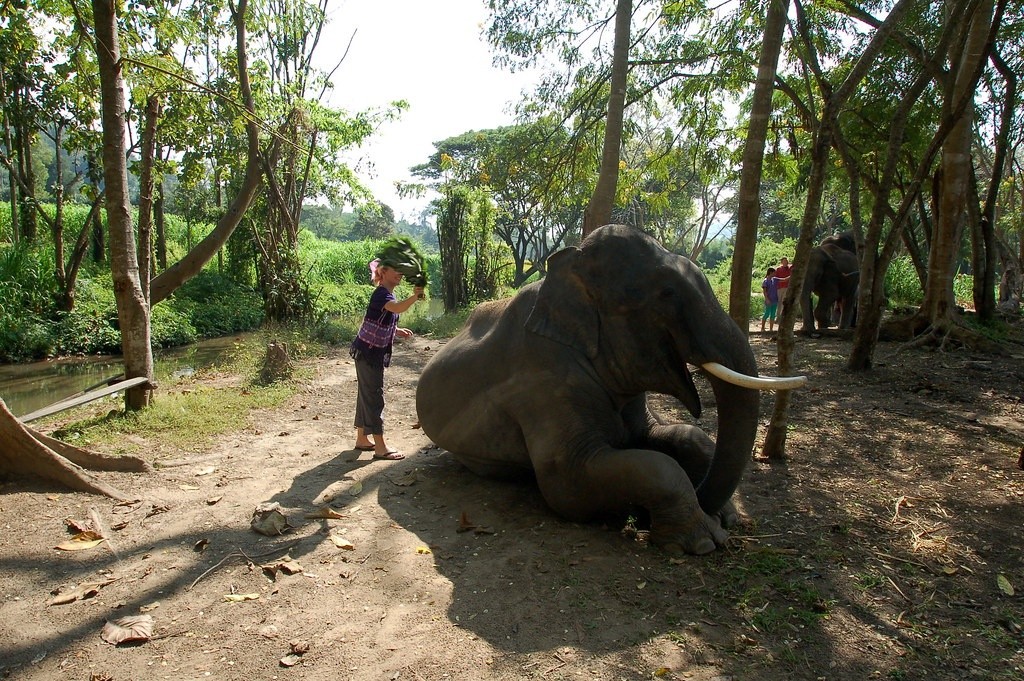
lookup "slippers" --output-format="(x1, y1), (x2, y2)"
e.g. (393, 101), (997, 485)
(373, 450), (405, 459)
(355, 444), (375, 450)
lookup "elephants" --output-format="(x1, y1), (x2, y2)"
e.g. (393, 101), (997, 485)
(799, 229), (859, 334)
(416, 223), (809, 556)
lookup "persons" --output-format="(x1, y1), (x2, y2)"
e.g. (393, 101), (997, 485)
(349, 258), (424, 460)
(774, 257), (794, 323)
(761, 268), (791, 331)
